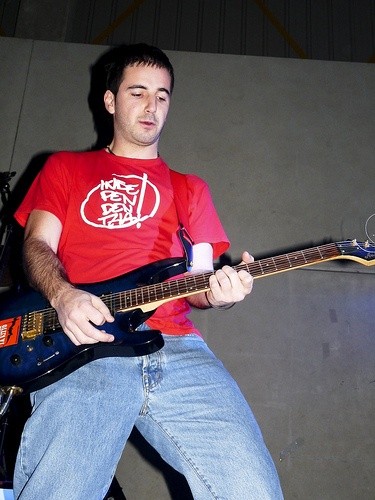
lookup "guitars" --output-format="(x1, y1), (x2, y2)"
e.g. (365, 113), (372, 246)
(0, 237), (375, 394)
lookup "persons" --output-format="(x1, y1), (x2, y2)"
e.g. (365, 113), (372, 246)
(13, 43), (284, 499)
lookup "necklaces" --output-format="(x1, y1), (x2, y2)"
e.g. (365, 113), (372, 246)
(106, 144), (160, 159)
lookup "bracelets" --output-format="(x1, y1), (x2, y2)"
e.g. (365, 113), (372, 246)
(205, 292), (236, 311)
